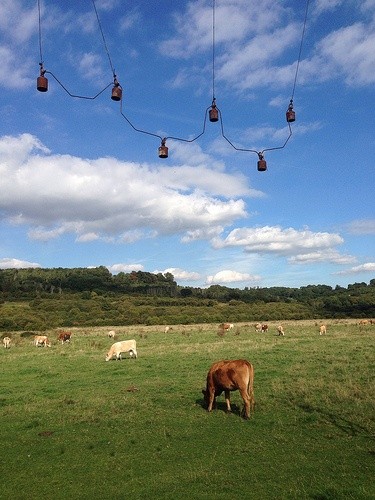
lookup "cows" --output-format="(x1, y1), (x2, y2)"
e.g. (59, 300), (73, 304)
(3, 337), (12, 349)
(109, 331), (115, 338)
(202, 359), (255, 420)
(360, 319), (375, 326)
(56, 332), (72, 344)
(163, 325), (174, 334)
(319, 325), (327, 336)
(217, 323), (235, 332)
(104, 339), (137, 361)
(32, 335), (51, 348)
(253, 323), (285, 336)
